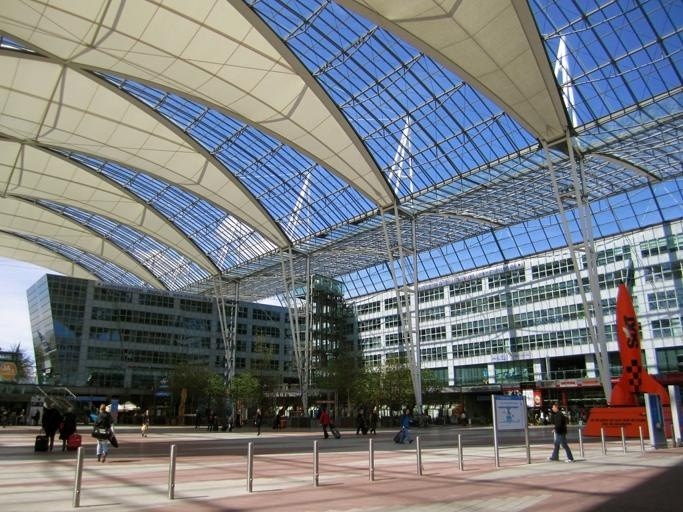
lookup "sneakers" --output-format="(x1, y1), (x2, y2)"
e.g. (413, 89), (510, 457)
(547, 456), (559, 462)
(565, 459), (574, 464)
(98, 454), (106, 463)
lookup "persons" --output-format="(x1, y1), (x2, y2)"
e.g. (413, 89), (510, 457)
(318, 406), (330, 439)
(369, 409), (378, 435)
(544, 403), (577, 463)
(193, 408), (241, 434)
(92, 403), (113, 464)
(253, 407), (263, 436)
(40, 400), (63, 452)
(141, 408), (151, 438)
(58, 405), (77, 451)
(397, 407), (415, 444)
(34, 409), (40, 425)
(355, 406), (367, 435)
(0, 406), (26, 428)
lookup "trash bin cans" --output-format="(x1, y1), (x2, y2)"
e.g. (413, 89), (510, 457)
(280, 416), (288, 429)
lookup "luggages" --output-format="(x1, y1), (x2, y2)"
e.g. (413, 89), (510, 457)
(67, 431), (80, 451)
(329, 423), (340, 438)
(35, 429), (48, 451)
(393, 429), (406, 443)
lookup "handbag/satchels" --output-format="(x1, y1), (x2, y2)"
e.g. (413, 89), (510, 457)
(92, 426), (110, 439)
(330, 424), (336, 429)
(141, 425), (147, 432)
(109, 428), (119, 448)
